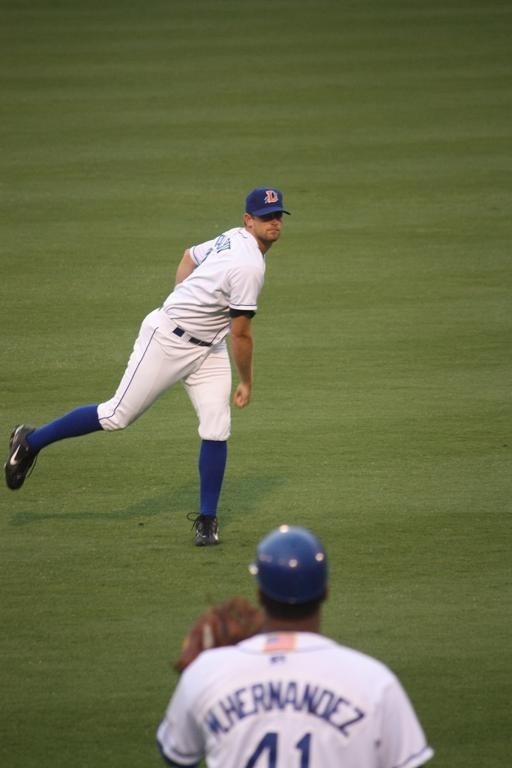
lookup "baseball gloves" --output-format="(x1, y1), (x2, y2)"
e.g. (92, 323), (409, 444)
(171, 597), (261, 673)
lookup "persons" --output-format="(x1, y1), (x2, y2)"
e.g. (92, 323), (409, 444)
(4, 187), (293, 546)
(154, 524), (437, 768)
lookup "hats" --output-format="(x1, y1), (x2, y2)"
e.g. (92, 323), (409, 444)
(246, 188), (290, 217)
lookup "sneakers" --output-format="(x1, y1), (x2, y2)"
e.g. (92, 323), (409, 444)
(4, 424), (38, 490)
(187, 512), (218, 545)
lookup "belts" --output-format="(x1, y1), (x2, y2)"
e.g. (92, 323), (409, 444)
(174, 327), (211, 346)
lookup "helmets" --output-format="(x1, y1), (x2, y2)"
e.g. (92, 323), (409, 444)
(257, 524), (327, 603)
